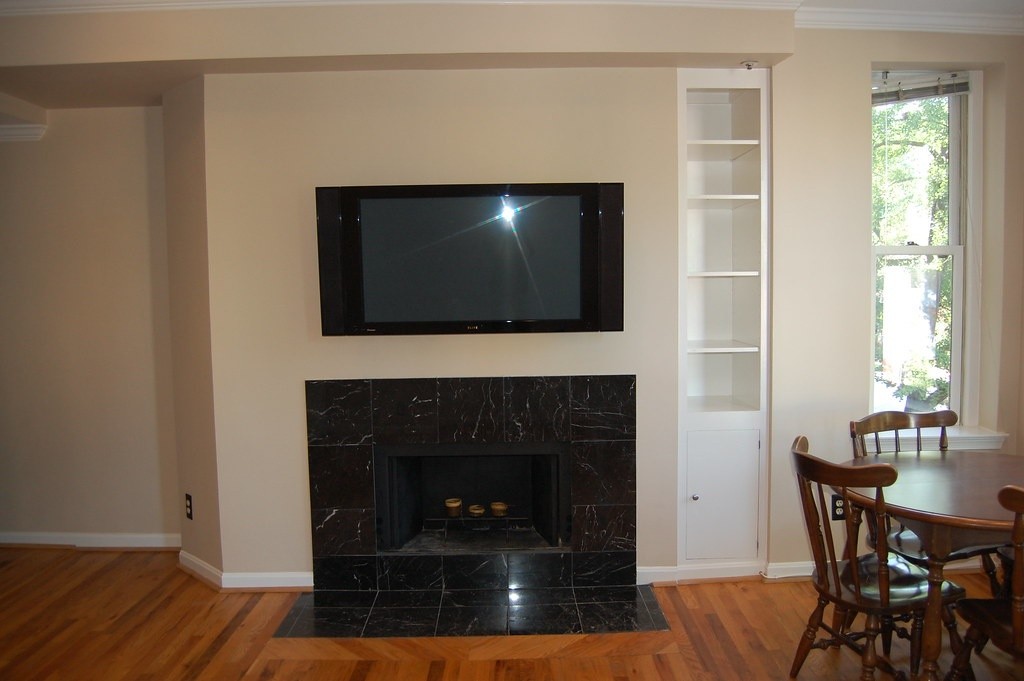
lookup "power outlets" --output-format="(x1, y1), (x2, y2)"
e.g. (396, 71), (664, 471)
(185, 493), (193, 520)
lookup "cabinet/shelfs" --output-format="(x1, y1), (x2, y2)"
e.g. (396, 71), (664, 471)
(687, 140), (761, 351)
(688, 427), (758, 562)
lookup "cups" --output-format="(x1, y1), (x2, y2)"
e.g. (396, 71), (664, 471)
(468, 505), (487, 517)
(445, 498), (461, 517)
(491, 502), (509, 517)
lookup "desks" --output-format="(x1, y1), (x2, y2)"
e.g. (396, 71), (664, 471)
(829, 449), (1024, 681)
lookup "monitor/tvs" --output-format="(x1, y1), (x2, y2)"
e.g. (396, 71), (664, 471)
(315, 182), (624, 336)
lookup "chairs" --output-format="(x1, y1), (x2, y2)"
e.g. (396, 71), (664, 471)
(849, 410), (1024, 681)
(950, 483), (1024, 681)
(790, 435), (977, 681)
